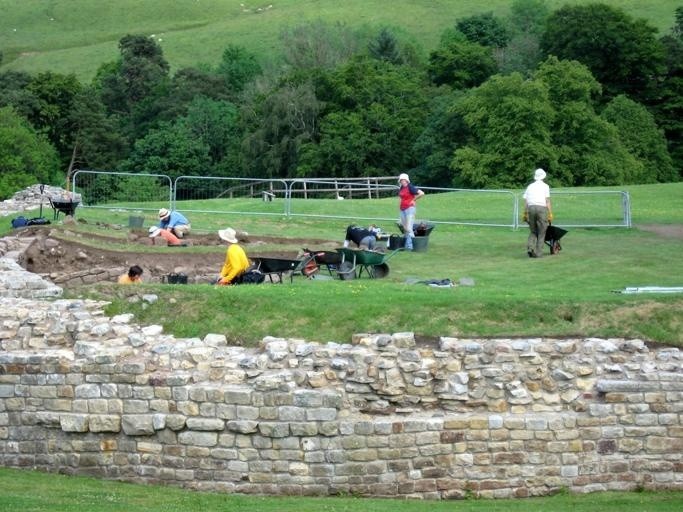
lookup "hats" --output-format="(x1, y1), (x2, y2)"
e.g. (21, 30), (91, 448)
(158, 208), (171, 220)
(534, 168), (547, 180)
(149, 226), (161, 238)
(218, 227), (238, 244)
(397, 173), (410, 184)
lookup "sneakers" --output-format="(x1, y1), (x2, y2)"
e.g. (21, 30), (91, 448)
(535, 254), (542, 258)
(528, 250), (535, 257)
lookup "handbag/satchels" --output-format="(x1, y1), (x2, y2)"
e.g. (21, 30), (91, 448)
(11, 216), (51, 228)
(231, 258), (265, 285)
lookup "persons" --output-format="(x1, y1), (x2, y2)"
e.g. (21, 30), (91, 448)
(217, 227), (250, 285)
(148, 225), (181, 244)
(344, 223), (377, 250)
(398, 173), (424, 251)
(523, 168), (552, 258)
(159, 208), (191, 239)
(118, 266), (143, 283)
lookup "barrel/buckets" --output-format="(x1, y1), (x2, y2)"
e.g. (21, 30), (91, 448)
(411, 236), (427, 251)
(129, 215), (145, 228)
(390, 233), (407, 250)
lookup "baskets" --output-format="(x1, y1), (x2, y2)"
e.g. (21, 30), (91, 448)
(387, 233), (406, 251)
(161, 271), (188, 285)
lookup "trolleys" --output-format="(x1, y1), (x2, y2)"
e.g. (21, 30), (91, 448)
(523, 215), (568, 255)
(45, 195), (80, 219)
(246, 247), (412, 285)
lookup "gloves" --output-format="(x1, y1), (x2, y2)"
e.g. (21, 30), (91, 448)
(547, 211), (553, 223)
(522, 210), (529, 222)
